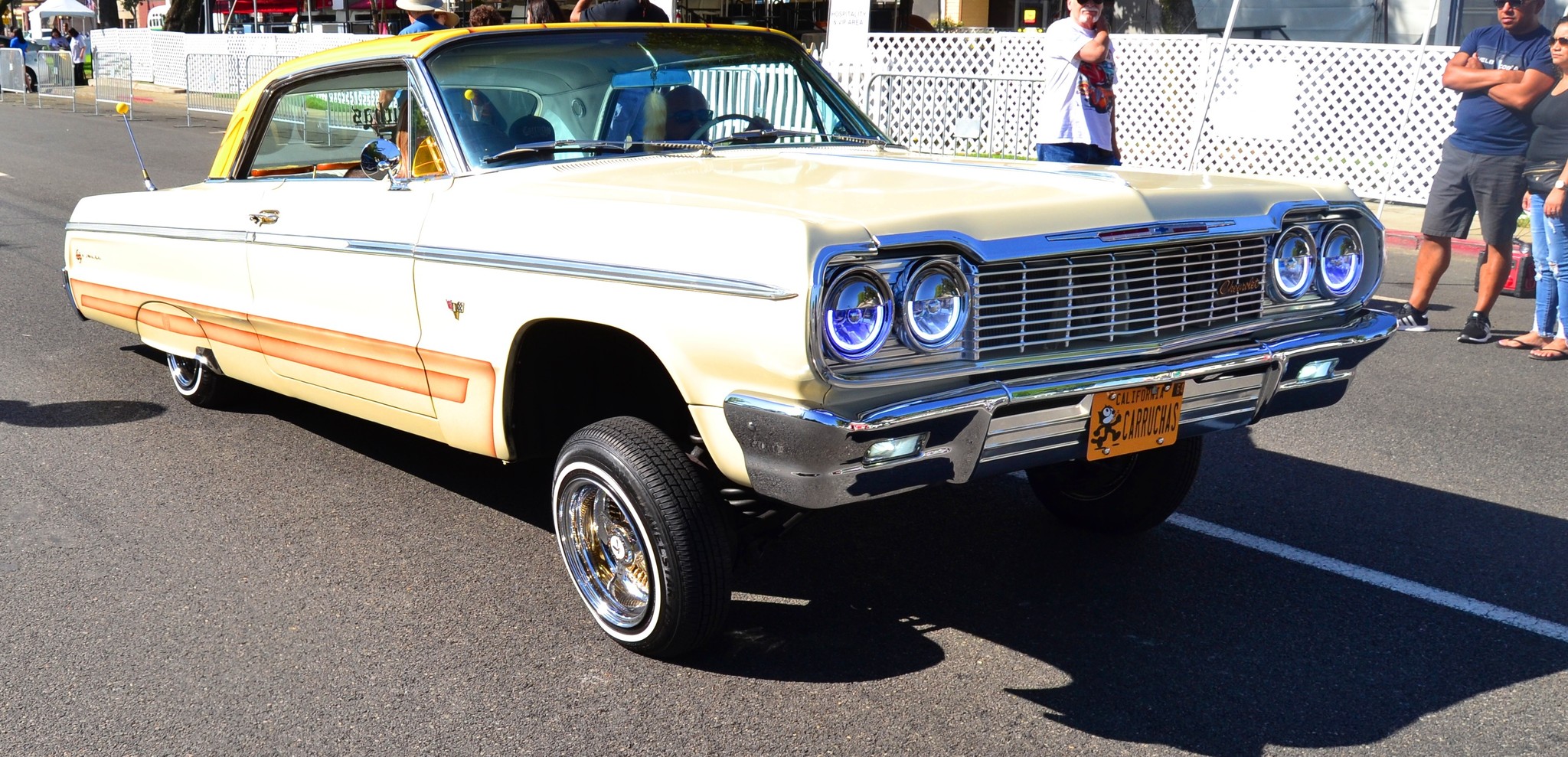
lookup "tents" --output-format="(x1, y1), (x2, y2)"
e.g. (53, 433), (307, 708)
(347, 0), (401, 10)
(28, 0), (95, 35)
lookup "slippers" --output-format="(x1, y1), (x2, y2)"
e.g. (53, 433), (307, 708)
(1496, 336), (1535, 350)
(1528, 347), (1568, 361)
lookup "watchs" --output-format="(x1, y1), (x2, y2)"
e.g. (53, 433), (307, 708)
(1555, 180), (1568, 191)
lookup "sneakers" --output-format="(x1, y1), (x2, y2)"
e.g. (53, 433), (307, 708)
(1390, 302), (1430, 332)
(1458, 311), (1492, 344)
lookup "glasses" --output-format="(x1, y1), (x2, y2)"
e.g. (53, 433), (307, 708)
(666, 109), (714, 125)
(1493, 0), (1524, 9)
(433, 14), (446, 19)
(1548, 36), (1568, 48)
(1077, 0), (1103, 4)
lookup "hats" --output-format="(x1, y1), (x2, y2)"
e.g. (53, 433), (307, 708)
(395, 0), (443, 12)
(434, 6), (460, 28)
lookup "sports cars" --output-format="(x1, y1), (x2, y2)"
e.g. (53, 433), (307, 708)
(63, 20), (1398, 649)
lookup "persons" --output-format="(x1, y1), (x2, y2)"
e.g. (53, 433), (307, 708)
(432, 0), (460, 29)
(469, 4), (505, 27)
(1035, 0), (1124, 167)
(49, 28), (72, 86)
(161, 0), (205, 34)
(626, 84), (780, 158)
(59, 22), (72, 41)
(1392, 0), (1563, 343)
(368, 0), (450, 139)
(506, 115), (558, 163)
(66, 28), (87, 87)
(526, 0), (560, 23)
(9, 27), (32, 94)
(1488, 15), (1568, 360)
(342, 94), (490, 179)
(569, 0), (670, 23)
